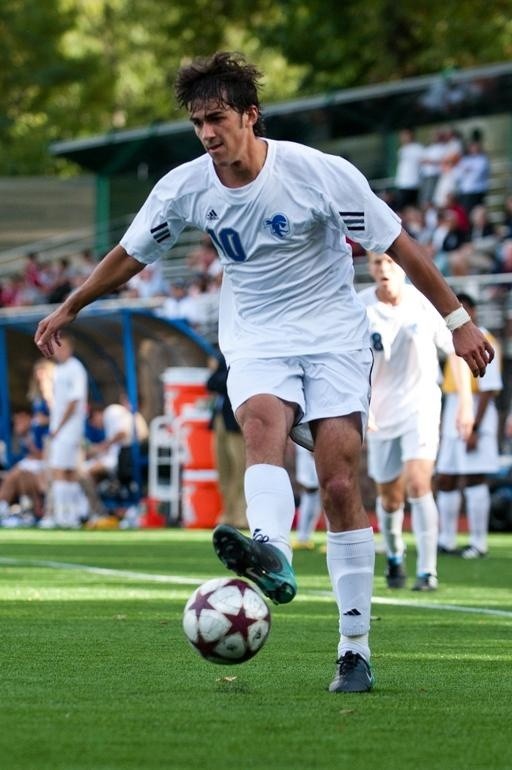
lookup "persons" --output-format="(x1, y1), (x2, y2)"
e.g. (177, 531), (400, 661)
(2, 118), (509, 594)
(29, 48), (500, 694)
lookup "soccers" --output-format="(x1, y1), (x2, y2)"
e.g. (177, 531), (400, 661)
(181, 578), (272, 666)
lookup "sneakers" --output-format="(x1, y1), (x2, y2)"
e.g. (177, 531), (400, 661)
(412, 572), (439, 591)
(439, 544), (487, 559)
(212, 522), (297, 604)
(385, 550), (407, 589)
(293, 539), (315, 550)
(328, 650), (374, 694)
